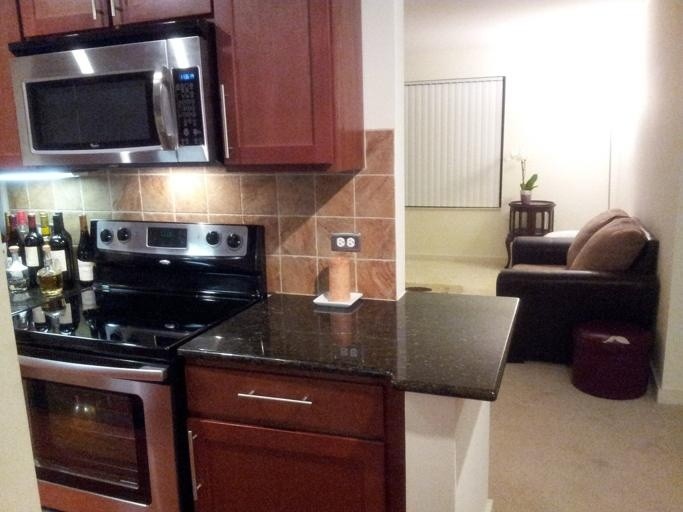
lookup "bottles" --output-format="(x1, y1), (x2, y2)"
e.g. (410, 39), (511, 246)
(13, 287), (102, 338)
(4, 209), (96, 295)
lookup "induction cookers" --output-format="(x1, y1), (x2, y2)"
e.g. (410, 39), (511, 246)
(12, 221), (261, 363)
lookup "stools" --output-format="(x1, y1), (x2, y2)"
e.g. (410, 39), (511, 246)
(574, 321), (652, 400)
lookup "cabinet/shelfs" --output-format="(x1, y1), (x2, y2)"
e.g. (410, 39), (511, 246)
(18, 0), (216, 38)
(0, 1), (24, 171)
(182, 363), (385, 511)
(213, 1), (365, 174)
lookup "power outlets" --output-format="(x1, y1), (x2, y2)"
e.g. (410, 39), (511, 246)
(331, 233), (362, 251)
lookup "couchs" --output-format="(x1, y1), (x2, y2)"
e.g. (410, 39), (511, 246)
(496, 208), (659, 362)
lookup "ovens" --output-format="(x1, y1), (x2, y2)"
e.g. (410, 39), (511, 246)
(16, 345), (195, 511)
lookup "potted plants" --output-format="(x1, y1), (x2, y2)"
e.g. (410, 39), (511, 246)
(520, 173), (537, 204)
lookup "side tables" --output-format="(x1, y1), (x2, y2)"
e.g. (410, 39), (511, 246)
(505, 200), (556, 268)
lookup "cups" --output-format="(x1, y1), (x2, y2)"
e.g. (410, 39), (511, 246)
(327, 255), (350, 302)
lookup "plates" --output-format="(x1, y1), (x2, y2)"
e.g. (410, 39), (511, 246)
(313, 291), (364, 308)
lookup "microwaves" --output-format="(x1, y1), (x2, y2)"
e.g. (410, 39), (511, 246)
(8, 34), (223, 167)
